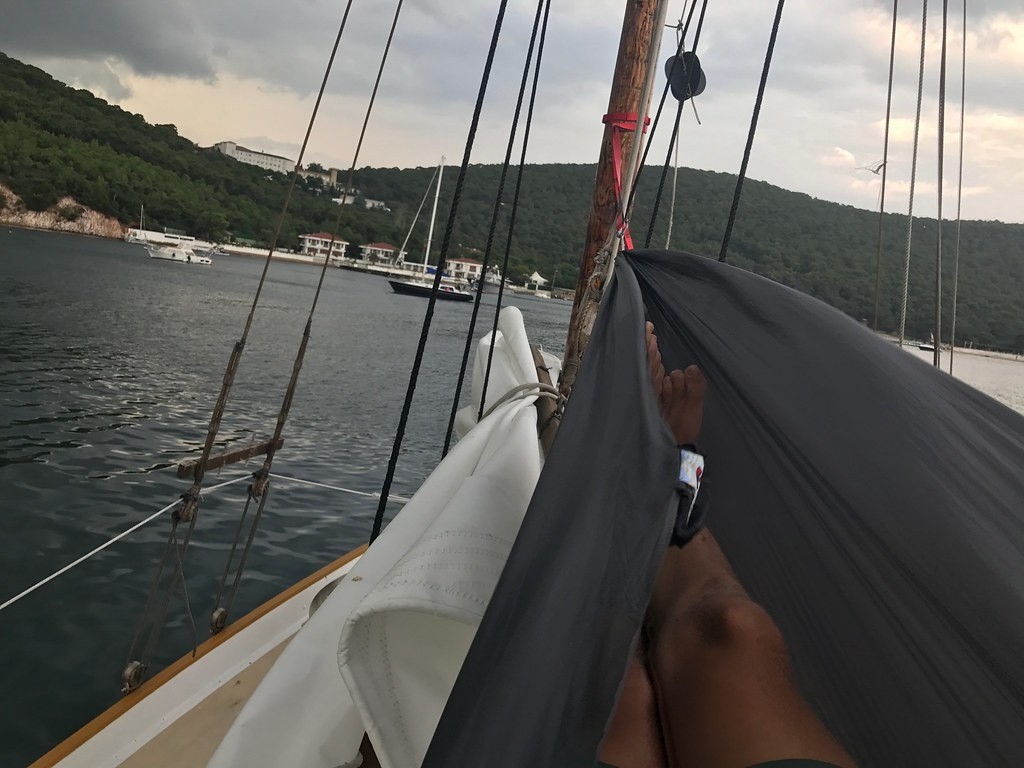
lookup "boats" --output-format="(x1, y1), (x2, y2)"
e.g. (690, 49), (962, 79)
(125, 204), (231, 264)
(917, 344), (942, 352)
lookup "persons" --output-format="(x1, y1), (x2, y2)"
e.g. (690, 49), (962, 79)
(595, 320), (863, 768)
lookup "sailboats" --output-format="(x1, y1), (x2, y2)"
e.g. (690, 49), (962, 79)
(382, 155), (476, 301)
